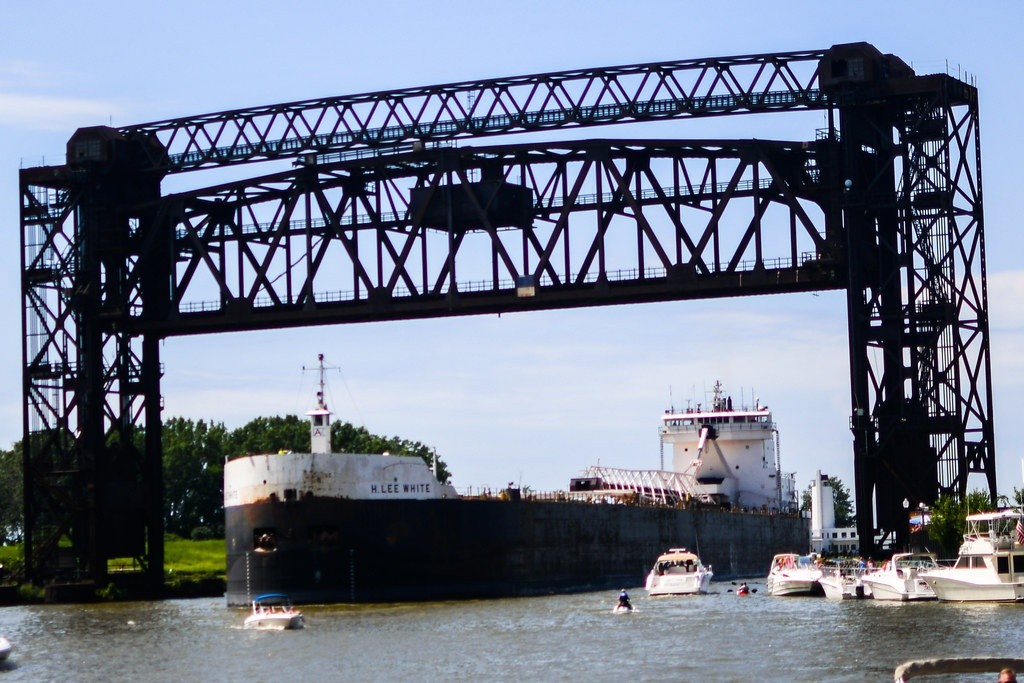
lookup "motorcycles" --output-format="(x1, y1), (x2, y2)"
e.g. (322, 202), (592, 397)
(737, 587), (750, 596)
(614, 597), (634, 614)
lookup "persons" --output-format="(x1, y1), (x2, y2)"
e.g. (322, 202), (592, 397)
(738, 582), (742, 587)
(866, 556), (874, 572)
(618, 588), (628, 602)
(998, 668), (1017, 683)
(815, 554), (865, 578)
(740, 582), (747, 590)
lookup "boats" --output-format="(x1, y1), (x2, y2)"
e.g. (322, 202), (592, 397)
(767, 552), (824, 597)
(861, 552), (946, 602)
(647, 548), (712, 597)
(244, 594), (305, 631)
(817, 559), (879, 601)
(917, 504), (1024, 602)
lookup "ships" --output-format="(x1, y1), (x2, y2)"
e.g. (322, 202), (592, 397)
(223, 379), (814, 608)
